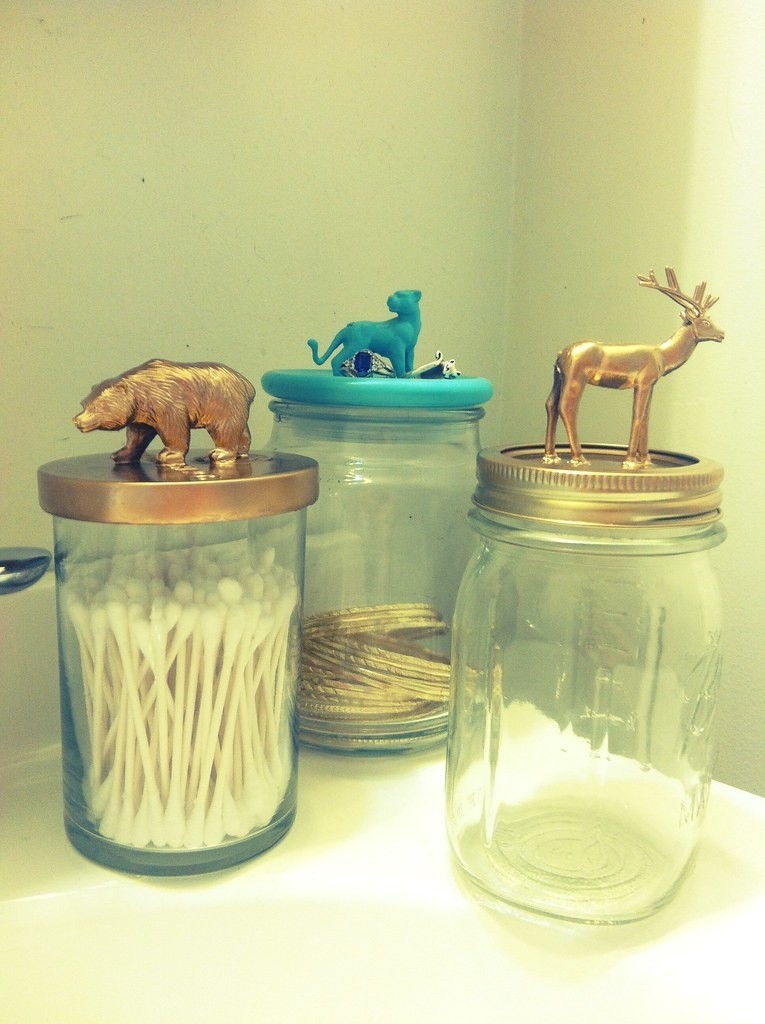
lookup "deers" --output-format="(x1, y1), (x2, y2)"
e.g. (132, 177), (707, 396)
(542, 265), (724, 471)
